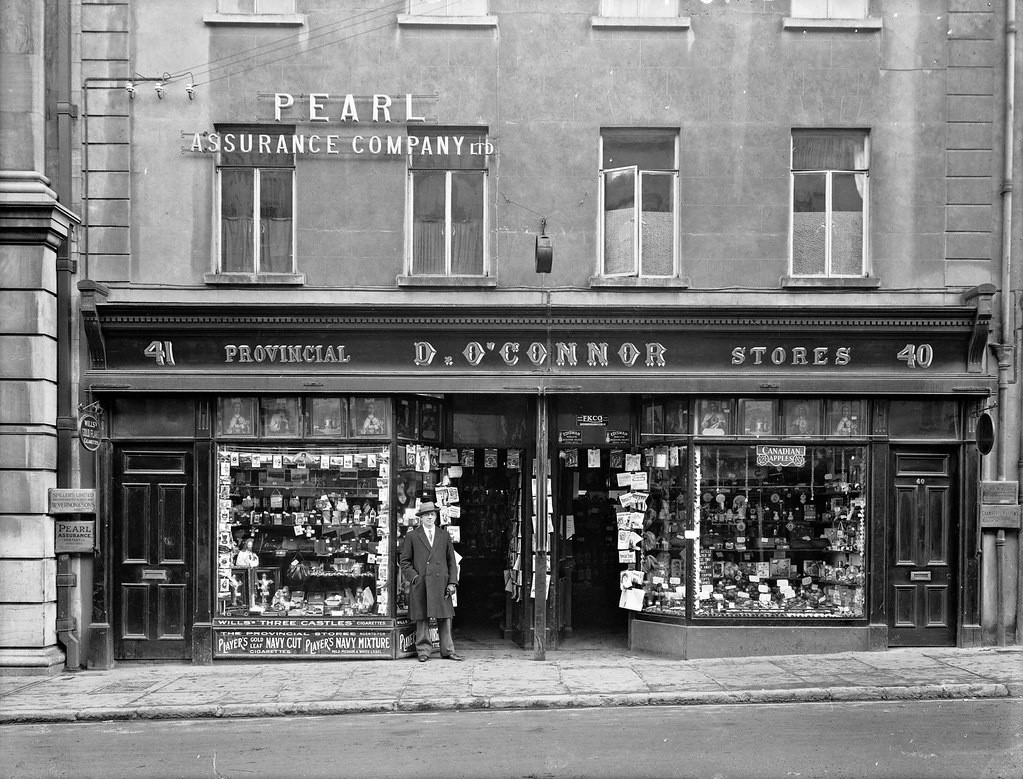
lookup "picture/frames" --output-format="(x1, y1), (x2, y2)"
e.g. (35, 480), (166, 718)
(636, 396), (664, 446)
(419, 396), (444, 443)
(303, 395), (349, 438)
(825, 393), (867, 439)
(349, 395), (392, 440)
(216, 395), (260, 439)
(736, 393), (780, 441)
(780, 393), (825, 439)
(664, 395), (691, 446)
(396, 396), (421, 443)
(260, 395), (303, 439)
(690, 394), (736, 441)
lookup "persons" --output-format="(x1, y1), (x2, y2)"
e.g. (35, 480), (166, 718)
(830, 403), (861, 439)
(359, 398), (383, 435)
(224, 398), (251, 435)
(396, 406), (416, 439)
(235, 538), (259, 568)
(268, 401), (295, 435)
(647, 409), (662, 441)
(701, 402), (728, 435)
(789, 403), (815, 439)
(400, 501), (466, 662)
(668, 402), (688, 439)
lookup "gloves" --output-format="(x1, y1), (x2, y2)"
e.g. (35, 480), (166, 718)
(447, 583), (456, 596)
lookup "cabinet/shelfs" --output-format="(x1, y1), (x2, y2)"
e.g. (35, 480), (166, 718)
(640, 444), (863, 620)
(221, 447), (420, 623)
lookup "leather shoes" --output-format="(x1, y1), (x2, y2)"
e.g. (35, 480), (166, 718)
(442, 653), (463, 660)
(418, 654), (427, 662)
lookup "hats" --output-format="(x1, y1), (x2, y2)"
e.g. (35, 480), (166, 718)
(414, 501), (440, 516)
